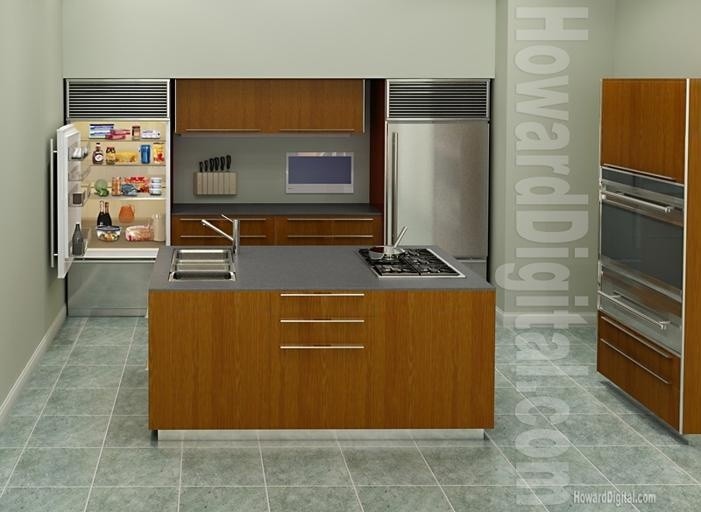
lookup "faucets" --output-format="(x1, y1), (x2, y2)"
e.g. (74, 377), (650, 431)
(200, 212), (241, 263)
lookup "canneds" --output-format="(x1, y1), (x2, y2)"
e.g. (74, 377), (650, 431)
(106, 147), (115, 165)
(132, 125), (140, 141)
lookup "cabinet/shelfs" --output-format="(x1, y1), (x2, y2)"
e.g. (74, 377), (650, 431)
(596, 310), (701, 435)
(599, 77), (701, 188)
(270, 291), (387, 429)
(171, 217), (275, 247)
(275, 217), (384, 246)
(175, 79), (364, 135)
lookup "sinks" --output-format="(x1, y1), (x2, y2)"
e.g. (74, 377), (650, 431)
(170, 271), (237, 284)
(171, 244), (233, 260)
(169, 260), (235, 271)
